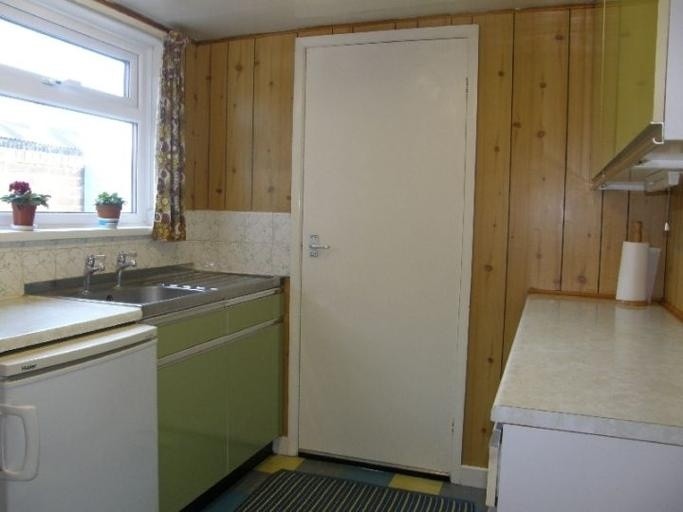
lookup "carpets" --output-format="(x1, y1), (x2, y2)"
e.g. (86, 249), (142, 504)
(226, 468), (473, 512)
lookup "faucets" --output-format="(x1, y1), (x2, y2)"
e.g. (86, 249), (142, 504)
(113, 248), (138, 290)
(80, 250), (106, 293)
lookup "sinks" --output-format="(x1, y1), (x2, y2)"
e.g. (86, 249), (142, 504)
(60, 280), (207, 310)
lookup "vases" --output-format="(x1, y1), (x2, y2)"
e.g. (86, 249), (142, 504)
(11, 203), (38, 232)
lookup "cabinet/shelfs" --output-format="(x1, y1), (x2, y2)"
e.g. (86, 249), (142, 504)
(588, 1), (683, 187)
(143, 304), (230, 512)
(230, 292), (284, 470)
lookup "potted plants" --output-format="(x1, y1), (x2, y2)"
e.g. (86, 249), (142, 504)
(93, 191), (125, 221)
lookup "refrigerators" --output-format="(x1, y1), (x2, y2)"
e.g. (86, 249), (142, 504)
(0, 325), (158, 511)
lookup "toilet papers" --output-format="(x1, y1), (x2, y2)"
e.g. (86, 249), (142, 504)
(615, 240), (662, 304)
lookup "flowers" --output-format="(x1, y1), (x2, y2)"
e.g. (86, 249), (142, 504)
(2, 180), (51, 208)
